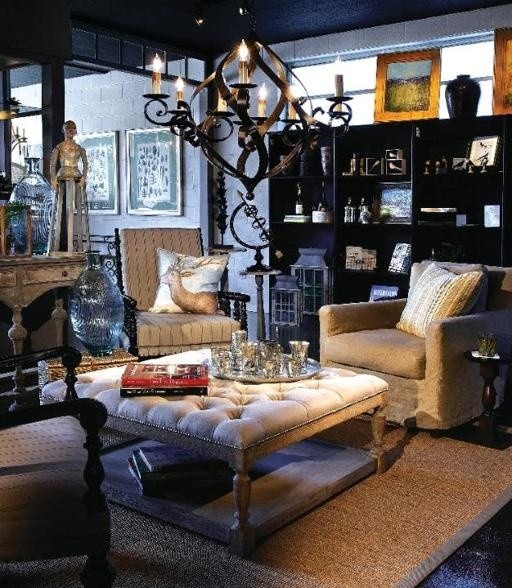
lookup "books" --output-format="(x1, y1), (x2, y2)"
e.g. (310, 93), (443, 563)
(388, 241), (411, 275)
(139, 445), (206, 473)
(120, 362), (209, 388)
(133, 449), (205, 480)
(420, 207), (457, 214)
(369, 284), (398, 301)
(127, 457), (198, 489)
(120, 385), (209, 398)
(283, 214), (310, 224)
(345, 242), (377, 270)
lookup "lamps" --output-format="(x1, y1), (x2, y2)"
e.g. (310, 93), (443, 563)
(144, 0), (352, 202)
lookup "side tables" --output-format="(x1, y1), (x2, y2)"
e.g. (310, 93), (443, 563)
(463, 349), (512, 437)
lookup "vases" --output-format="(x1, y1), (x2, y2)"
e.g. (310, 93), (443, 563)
(8, 157), (61, 256)
(444, 74), (480, 118)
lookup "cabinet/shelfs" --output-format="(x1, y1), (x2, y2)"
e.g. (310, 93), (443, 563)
(263, 114), (512, 326)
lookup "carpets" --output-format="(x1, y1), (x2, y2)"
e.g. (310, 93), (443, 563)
(0, 431), (512, 588)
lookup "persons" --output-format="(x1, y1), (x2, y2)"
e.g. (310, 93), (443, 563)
(50, 121), (88, 189)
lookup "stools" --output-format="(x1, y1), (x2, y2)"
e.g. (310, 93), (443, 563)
(39, 349), (138, 400)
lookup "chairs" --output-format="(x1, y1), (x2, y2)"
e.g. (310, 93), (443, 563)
(111, 228), (250, 357)
(318, 261), (512, 430)
(0, 347), (115, 587)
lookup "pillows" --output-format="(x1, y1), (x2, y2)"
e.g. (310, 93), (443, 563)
(407, 263), (488, 313)
(147, 247), (230, 315)
(395, 264), (485, 338)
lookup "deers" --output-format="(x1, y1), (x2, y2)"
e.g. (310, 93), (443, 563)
(160, 256), (225, 314)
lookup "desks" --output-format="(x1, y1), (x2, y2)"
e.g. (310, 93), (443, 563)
(1, 252), (87, 411)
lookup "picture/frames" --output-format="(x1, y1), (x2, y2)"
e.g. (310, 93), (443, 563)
(493, 28), (512, 115)
(466, 135), (503, 169)
(125, 127), (183, 216)
(71, 130), (121, 216)
(373, 182), (412, 223)
(375, 48), (441, 123)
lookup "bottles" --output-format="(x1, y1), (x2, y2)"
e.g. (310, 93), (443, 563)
(8, 157), (53, 256)
(317, 181), (329, 212)
(295, 182), (306, 215)
(344, 197), (356, 224)
(358, 197), (372, 226)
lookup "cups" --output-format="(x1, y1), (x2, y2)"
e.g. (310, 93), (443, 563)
(241, 341), (258, 367)
(288, 340), (309, 374)
